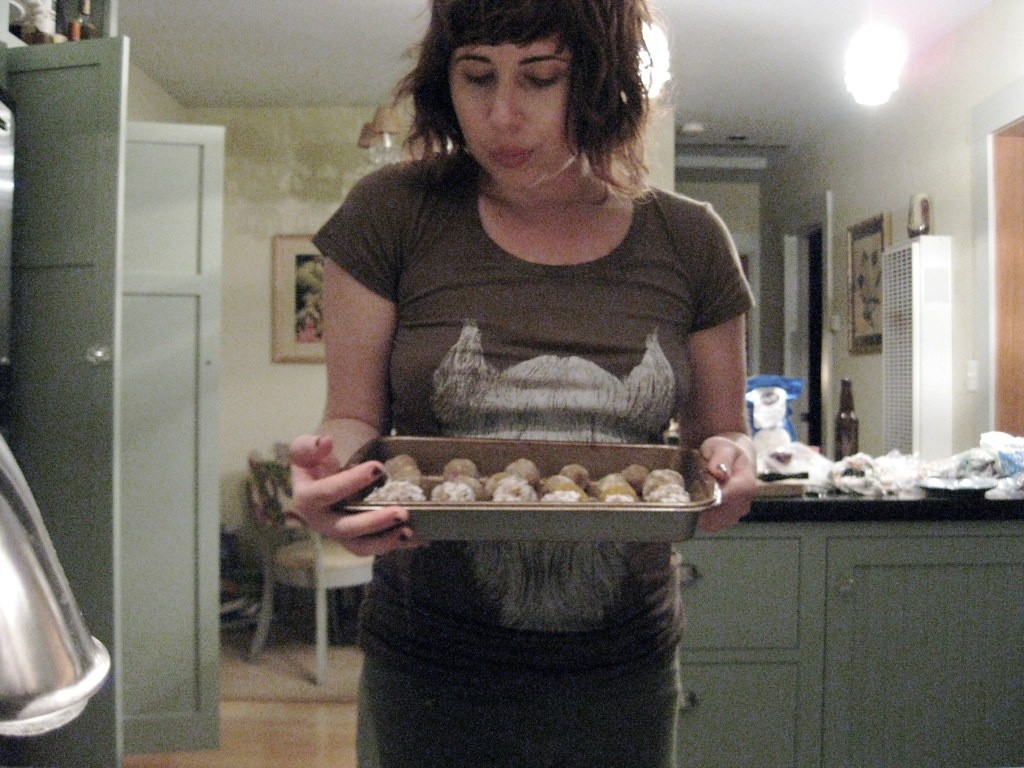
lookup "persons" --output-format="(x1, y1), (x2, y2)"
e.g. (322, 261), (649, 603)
(290, 0), (758, 768)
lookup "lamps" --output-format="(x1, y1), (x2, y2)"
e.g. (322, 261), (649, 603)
(358, 107), (400, 168)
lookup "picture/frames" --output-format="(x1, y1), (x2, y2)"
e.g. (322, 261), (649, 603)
(846, 212), (893, 355)
(270, 233), (326, 363)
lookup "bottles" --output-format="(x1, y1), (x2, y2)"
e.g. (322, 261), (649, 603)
(834, 377), (860, 476)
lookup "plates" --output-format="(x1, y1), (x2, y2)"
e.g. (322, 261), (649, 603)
(915, 477), (998, 495)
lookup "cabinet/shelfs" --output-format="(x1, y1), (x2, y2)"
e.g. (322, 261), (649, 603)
(672, 515), (1024, 768)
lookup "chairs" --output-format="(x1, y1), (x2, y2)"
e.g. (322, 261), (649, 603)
(246, 450), (375, 689)
